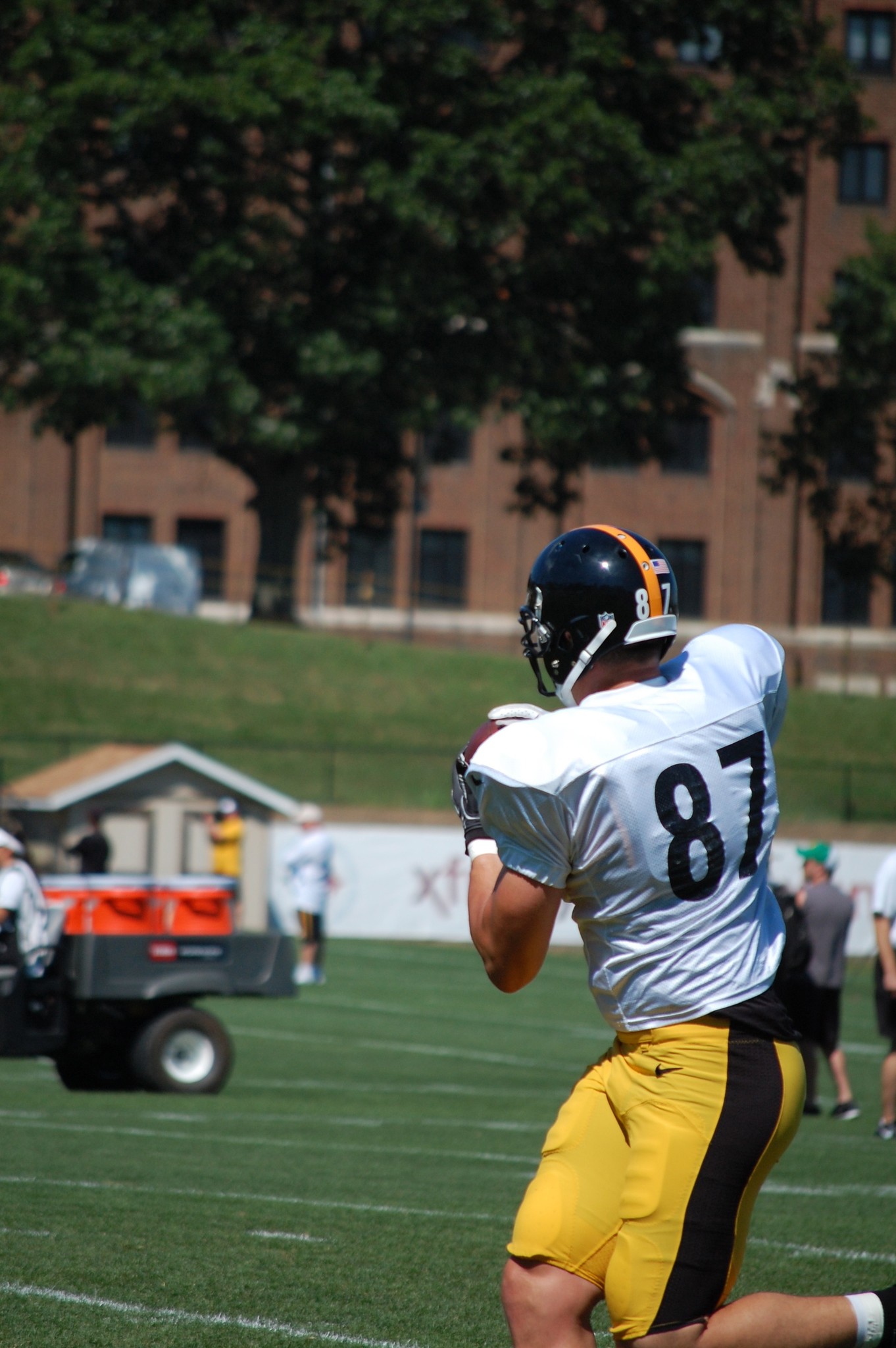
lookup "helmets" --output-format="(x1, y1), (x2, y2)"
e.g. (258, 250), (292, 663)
(520, 523), (681, 707)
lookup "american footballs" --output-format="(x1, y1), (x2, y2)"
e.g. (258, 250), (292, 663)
(463, 713), (527, 777)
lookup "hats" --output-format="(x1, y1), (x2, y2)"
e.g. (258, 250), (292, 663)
(218, 798), (236, 813)
(296, 802), (322, 822)
(797, 842), (839, 873)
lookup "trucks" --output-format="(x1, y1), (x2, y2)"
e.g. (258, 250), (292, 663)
(1, 933), (306, 1098)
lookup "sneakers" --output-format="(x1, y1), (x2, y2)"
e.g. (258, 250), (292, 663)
(833, 1102), (861, 1122)
(874, 1120), (893, 1139)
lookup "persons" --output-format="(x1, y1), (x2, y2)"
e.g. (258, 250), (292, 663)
(283, 802), (337, 987)
(871, 848), (896, 1142)
(766, 880), (812, 1041)
(794, 839), (861, 1120)
(60, 811), (110, 873)
(452, 522), (896, 1348)
(0, 809), (48, 967)
(202, 796), (245, 928)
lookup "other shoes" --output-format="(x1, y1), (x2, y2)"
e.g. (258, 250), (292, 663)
(802, 1102), (821, 1118)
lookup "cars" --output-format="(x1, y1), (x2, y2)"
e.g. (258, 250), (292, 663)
(2, 548), (70, 602)
